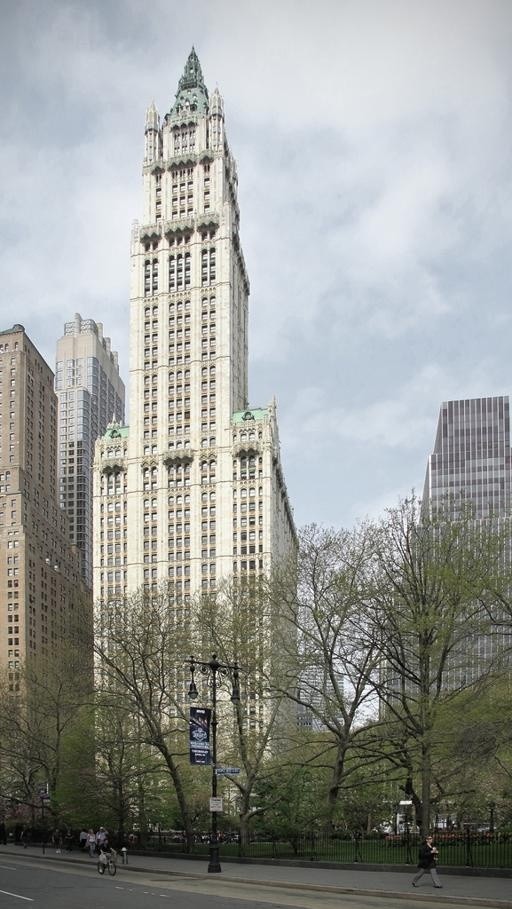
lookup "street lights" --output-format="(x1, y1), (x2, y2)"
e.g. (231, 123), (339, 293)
(184, 655), (243, 873)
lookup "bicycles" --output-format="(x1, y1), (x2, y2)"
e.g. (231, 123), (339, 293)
(98, 852), (118, 875)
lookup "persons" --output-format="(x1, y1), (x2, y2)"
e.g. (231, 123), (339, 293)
(51, 828), (61, 854)
(411, 833), (443, 888)
(63, 829), (75, 853)
(79, 826), (118, 866)
(175, 831), (225, 844)
(20, 828), (28, 848)
